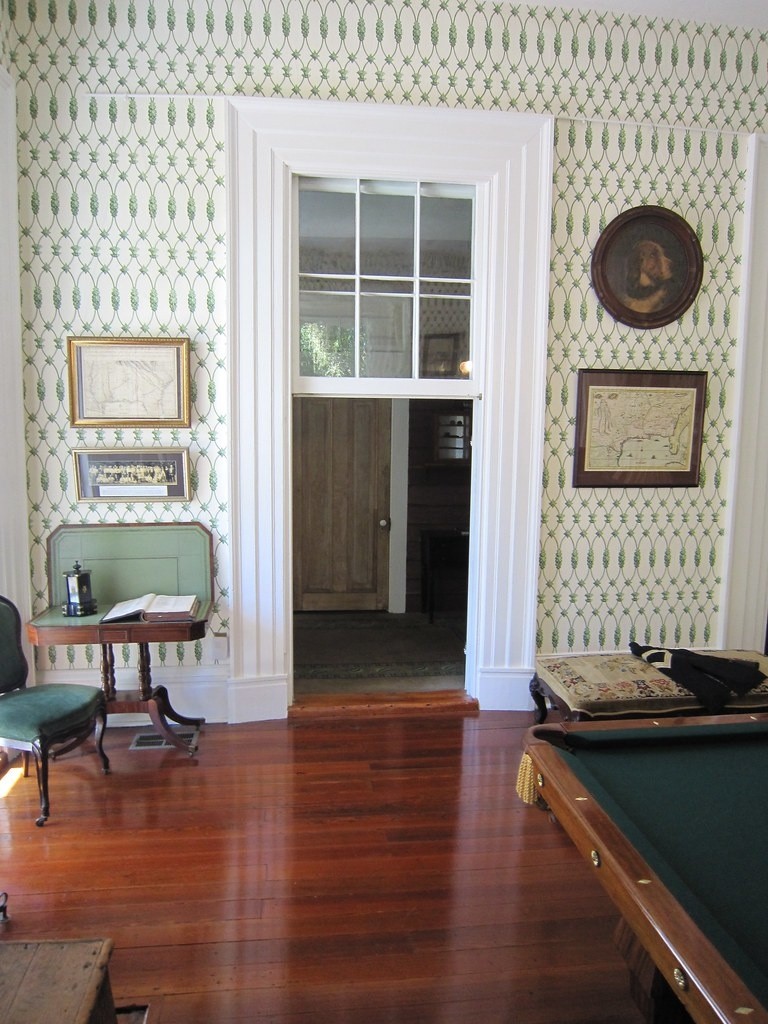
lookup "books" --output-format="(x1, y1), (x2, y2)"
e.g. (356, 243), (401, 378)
(100, 592), (201, 624)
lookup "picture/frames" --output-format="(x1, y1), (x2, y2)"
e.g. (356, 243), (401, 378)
(65, 334), (191, 429)
(69, 446), (190, 503)
(572, 367), (708, 487)
(589, 201), (703, 330)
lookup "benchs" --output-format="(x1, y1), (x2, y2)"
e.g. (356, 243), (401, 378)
(528, 648), (767, 723)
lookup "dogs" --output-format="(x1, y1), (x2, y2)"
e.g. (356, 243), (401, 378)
(620, 238), (679, 312)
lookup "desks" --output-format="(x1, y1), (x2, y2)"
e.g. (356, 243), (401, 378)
(518, 710), (767, 1024)
(28, 520), (215, 756)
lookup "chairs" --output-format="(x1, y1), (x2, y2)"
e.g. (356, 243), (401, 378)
(0, 594), (111, 826)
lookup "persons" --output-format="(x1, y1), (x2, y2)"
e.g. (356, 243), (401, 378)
(89, 462), (175, 485)
